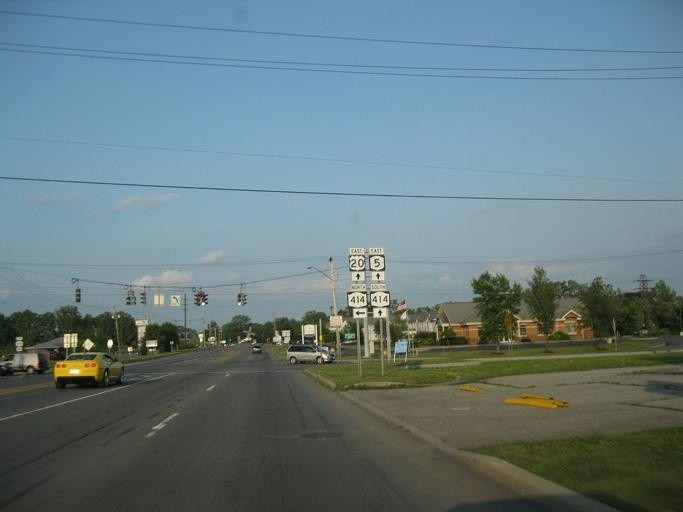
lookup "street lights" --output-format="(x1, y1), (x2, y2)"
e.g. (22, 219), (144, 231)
(307, 266), (342, 363)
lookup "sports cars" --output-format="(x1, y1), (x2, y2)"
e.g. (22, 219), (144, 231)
(53, 352), (126, 389)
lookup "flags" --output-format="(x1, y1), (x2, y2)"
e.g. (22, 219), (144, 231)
(395, 298), (405, 311)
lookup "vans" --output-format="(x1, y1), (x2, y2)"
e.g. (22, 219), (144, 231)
(287, 344), (336, 364)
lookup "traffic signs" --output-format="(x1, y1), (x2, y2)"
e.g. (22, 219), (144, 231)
(347, 246), (391, 308)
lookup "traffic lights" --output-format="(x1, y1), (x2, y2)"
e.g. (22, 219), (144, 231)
(125, 291), (146, 305)
(193, 292), (209, 307)
(236, 292), (247, 307)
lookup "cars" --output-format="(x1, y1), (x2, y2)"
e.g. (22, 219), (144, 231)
(0, 364), (13, 375)
(252, 345), (262, 353)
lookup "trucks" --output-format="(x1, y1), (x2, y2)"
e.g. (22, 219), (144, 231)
(0, 352), (49, 374)
